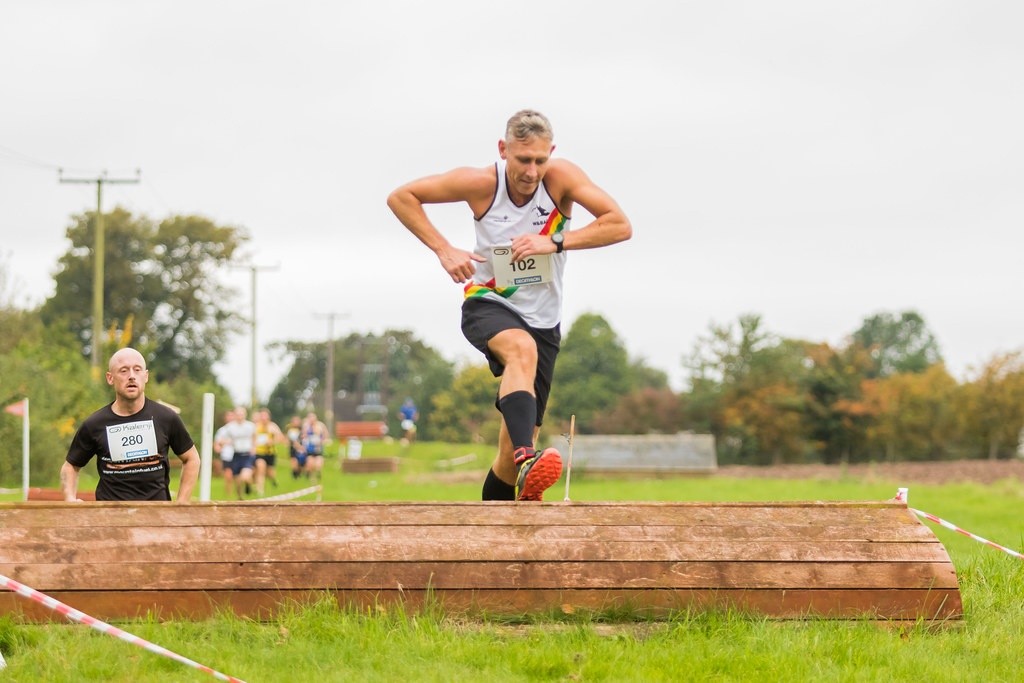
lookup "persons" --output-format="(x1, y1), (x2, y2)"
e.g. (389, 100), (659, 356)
(60, 347), (200, 501)
(387, 111), (632, 502)
(396, 397), (420, 444)
(214, 407), (329, 500)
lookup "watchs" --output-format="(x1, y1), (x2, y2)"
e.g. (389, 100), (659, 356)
(552, 232), (564, 253)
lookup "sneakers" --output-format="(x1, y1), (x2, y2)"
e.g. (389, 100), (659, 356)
(514, 446), (562, 502)
